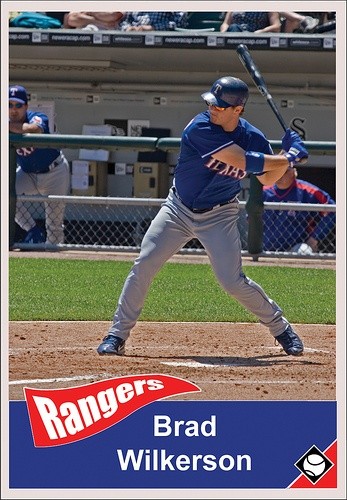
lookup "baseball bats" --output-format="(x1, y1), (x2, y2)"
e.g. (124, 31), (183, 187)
(236, 44), (308, 164)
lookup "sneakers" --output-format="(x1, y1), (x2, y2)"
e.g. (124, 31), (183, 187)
(22, 228), (44, 243)
(97, 335), (125, 355)
(273, 324), (303, 355)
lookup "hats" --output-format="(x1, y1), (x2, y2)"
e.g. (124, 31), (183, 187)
(9, 85), (27, 105)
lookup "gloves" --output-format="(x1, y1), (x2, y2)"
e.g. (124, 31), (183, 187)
(280, 126), (301, 153)
(283, 141), (308, 167)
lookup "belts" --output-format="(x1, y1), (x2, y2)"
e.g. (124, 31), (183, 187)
(20, 160), (58, 174)
(171, 187), (235, 214)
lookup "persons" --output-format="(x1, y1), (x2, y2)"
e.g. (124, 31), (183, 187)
(9, 84), (71, 249)
(218, 12), (280, 33)
(97, 75), (309, 358)
(120, 12), (192, 31)
(66, 12), (125, 31)
(279, 12), (328, 34)
(237, 163), (333, 254)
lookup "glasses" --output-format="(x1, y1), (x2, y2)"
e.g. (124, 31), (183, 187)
(205, 101), (226, 111)
(9, 102), (23, 108)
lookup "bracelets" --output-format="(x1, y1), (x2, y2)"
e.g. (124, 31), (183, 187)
(244, 151), (264, 173)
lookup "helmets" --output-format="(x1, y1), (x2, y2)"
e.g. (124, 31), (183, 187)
(200, 76), (248, 108)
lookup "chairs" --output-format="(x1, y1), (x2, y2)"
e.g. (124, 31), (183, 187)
(184, 12), (225, 32)
